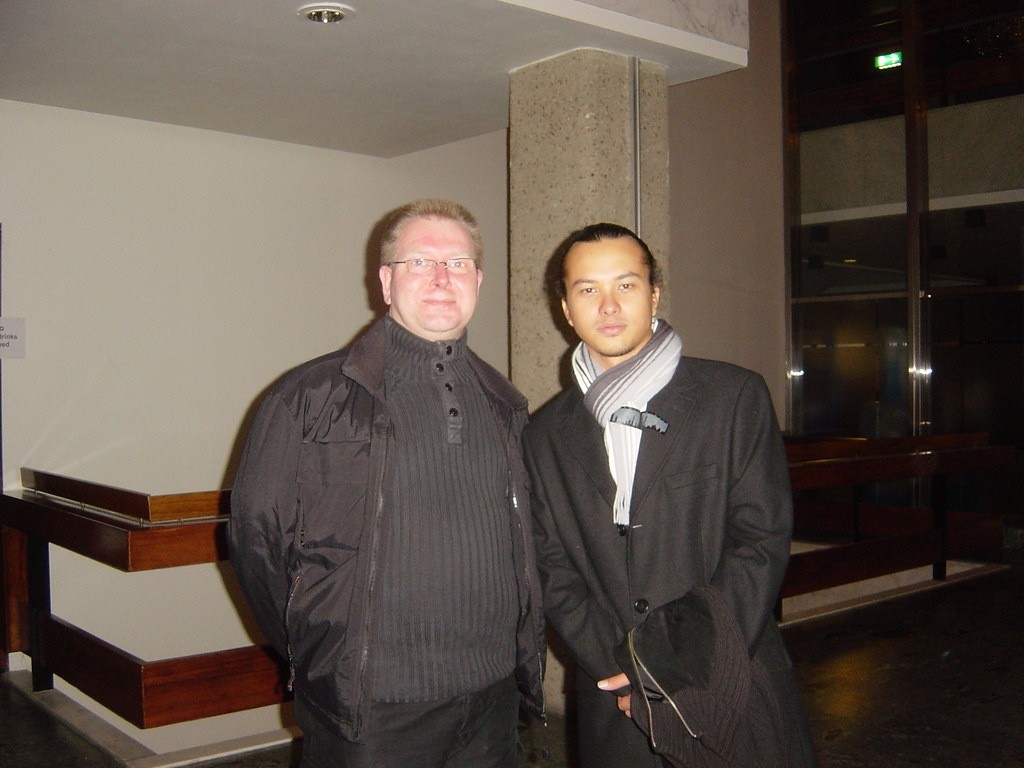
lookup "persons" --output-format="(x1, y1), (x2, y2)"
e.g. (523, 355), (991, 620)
(520, 223), (819, 768)
(228, 199), (553, 767)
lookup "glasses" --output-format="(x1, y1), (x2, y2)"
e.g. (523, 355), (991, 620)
(389, 257), (484, 276)
